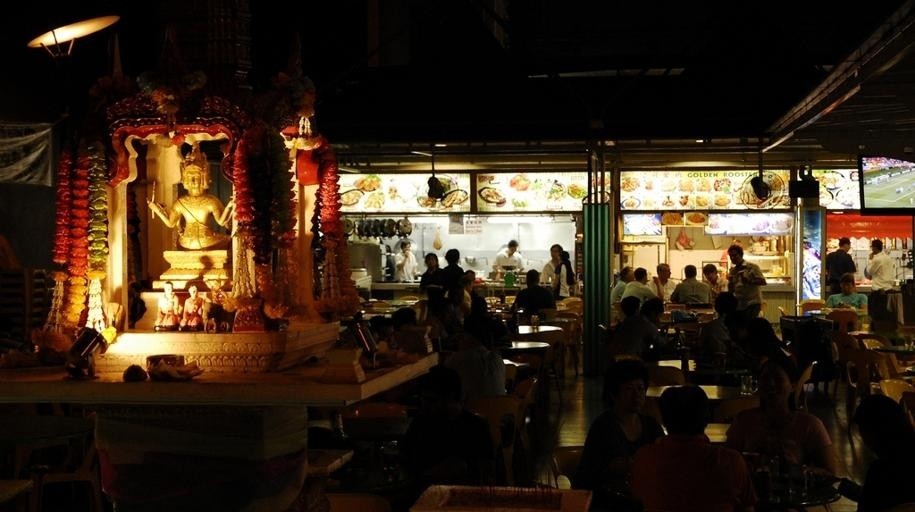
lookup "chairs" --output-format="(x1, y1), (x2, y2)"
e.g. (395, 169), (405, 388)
(326, 282), (915, 512)
(36, 426), (102, 511)
(1, 470), (39, 512)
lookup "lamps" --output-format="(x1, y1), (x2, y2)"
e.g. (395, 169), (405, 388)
(428, 155), (443, 199)
(750, 150), (771, 200)
(21, 0), (120, 59)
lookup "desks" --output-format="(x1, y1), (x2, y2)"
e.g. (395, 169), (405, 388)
(1, 344), (441, 512)
(1, 415), (96, 473)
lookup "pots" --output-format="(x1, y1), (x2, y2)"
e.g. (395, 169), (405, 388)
(340, 213), (413, 238)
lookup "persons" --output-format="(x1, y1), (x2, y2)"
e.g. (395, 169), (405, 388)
(146, 143), (233, 251)
(155, 282), (178, 326)
(581, 245), (836, 512)
(180, 286), (204, 327)
(366, 238), (579, 512)
(824, 237), (897, 325)
(121, 364), (148, 382)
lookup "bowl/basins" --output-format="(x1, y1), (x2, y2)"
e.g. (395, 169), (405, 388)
(510, 175), (530, 191)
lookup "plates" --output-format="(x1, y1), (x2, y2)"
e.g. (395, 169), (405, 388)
(661, 212), (708, 226)
(740, 171), (785, 211)
(818, 170), (860, 208)
(581, 190), (611, 204)
(621, 176), (733, 209)
(418, 174), (459, 210)
(478, 187), (508, 204)
(705, 214), (795, 233)
(442, 189), (467, 207)
(340, 189), (363, 206)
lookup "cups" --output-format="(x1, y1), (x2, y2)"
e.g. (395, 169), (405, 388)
(531, 315), (540, 328)
(905, 340), (913, 351)
(532, 328), (538, 333)
(739, 373), (759, 396)
